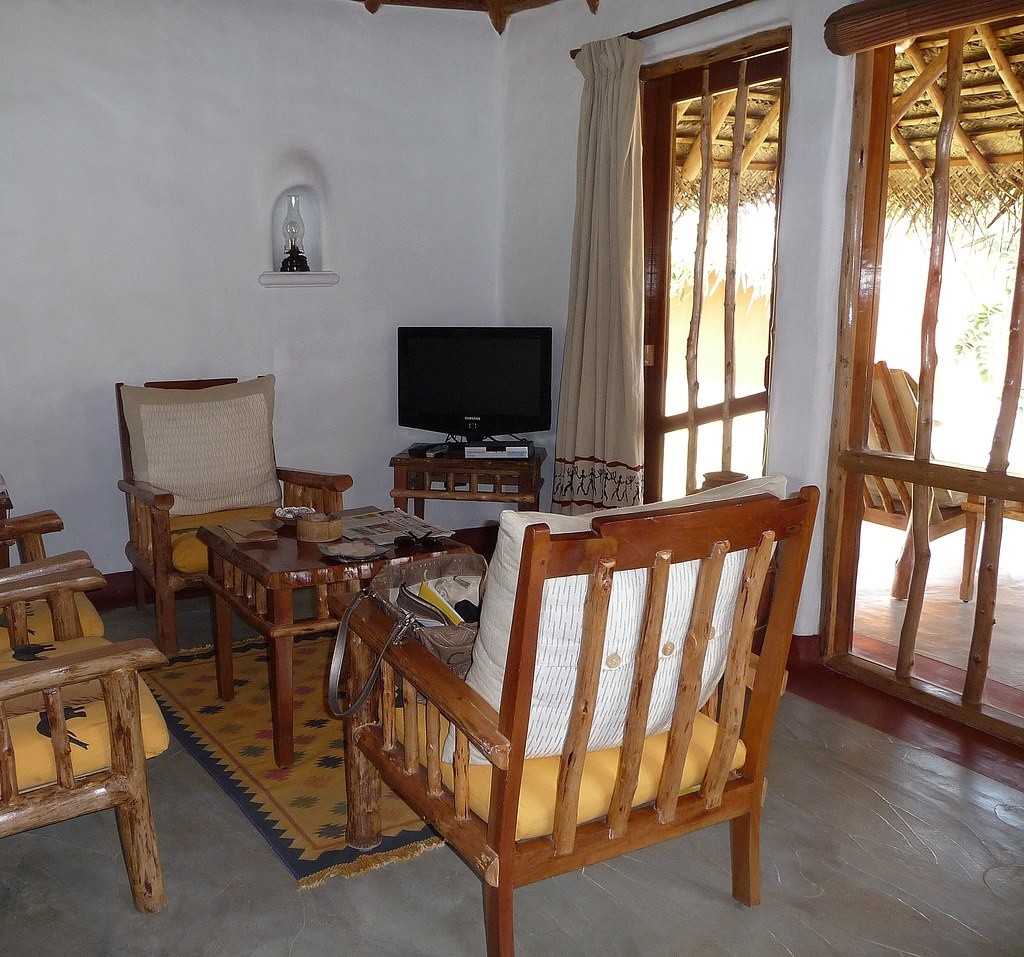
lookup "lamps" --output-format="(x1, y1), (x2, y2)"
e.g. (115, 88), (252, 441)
(279, 195), (312, 272)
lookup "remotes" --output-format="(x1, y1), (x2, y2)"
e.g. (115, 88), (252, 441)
(426, 446), (448, 458)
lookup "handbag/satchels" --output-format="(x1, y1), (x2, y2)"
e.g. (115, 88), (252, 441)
(327, 553), (488, 720)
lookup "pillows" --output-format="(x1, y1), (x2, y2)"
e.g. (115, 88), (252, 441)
(119, 374), (282, 517)
(435, 474), (787, 766)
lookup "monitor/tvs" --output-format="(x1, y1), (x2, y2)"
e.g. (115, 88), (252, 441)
(396, 326), (552, 447)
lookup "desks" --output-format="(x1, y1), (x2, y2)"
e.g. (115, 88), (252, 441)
(959, 491), (1024, 603)
(388, 442), (548, 521)
(195, 505), (478, 772)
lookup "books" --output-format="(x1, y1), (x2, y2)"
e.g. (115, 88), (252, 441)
(419, 581), (465, 626)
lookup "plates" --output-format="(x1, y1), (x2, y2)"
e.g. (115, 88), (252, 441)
(318, 539), (389, 562)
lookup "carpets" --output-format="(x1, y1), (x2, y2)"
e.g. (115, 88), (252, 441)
(137, 632), (446, 892)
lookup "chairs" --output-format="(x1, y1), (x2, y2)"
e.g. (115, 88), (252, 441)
(325, 472), (822, 957)
(862, 360), (984, 602)
(0, 488), (171, 916)
(114, 374), (356, 658)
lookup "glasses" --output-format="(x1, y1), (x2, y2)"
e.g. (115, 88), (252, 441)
(395, 531), (444, 549)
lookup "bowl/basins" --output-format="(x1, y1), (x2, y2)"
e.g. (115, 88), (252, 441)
(274, 507), (317, 525)
